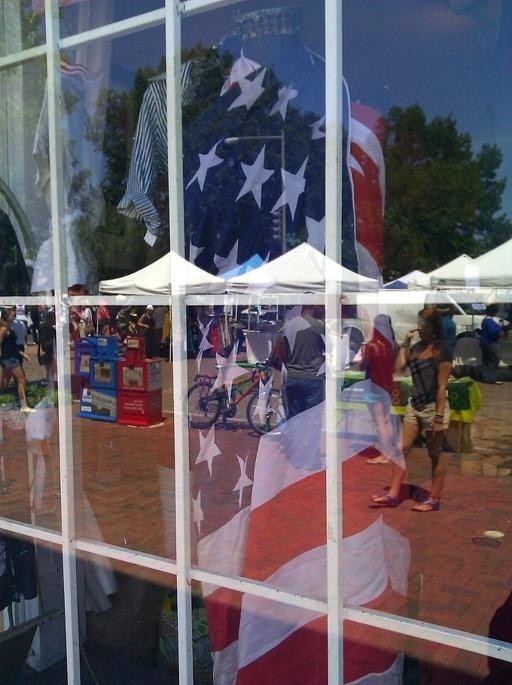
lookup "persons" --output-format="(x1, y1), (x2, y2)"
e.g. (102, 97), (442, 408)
(357, 313), (396, 466)
(478, 304), (504, 385)
(1, 304), (167, 417)
(367, 307), (453, 513)
(266, 305), (323, 471)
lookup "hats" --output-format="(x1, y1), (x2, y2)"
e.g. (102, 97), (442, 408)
(145, 305), (155, 311)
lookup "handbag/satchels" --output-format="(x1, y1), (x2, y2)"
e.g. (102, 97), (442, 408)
(78, 321), (94, 338)
(38, 354), (47, 365)
(135, 324), (149, 334)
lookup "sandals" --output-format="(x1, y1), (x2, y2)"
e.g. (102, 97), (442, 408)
(370, 493), (401, 507)
(365, 454), (391, 466)
(410, 500), (442, 512)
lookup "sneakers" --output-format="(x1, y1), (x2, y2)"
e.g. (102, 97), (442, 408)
(308, 461), (329, 470)
(292, 461), (307, 468)
(19, 407), (36, 412)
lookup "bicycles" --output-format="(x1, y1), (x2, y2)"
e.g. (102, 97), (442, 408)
(182, 359), (291, 441)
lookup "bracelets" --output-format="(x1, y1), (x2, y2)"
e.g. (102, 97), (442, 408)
(433, 414), (443, 423)
(402, 345), (408, 350)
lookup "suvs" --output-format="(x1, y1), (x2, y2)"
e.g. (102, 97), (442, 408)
(324, 287), (512, 384)
